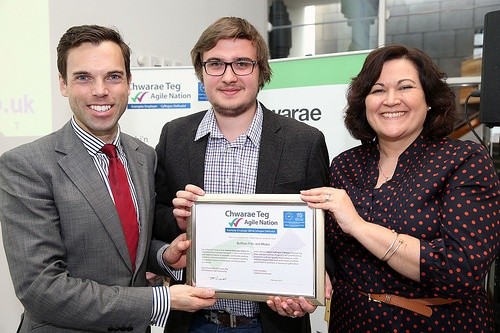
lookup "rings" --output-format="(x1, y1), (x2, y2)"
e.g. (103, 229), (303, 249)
(326, 193), (331, 202)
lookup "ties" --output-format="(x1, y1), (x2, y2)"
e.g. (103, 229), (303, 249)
(98, 144), (141, 273)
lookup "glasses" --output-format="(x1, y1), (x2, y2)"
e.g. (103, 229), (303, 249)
(200, 58), (259, 77)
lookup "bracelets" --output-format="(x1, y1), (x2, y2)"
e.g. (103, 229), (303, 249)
(380, 229), (404, 262)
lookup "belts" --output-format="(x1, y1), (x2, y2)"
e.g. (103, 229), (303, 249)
(359, 292), (462, 318)
(198, 309), (259, 328)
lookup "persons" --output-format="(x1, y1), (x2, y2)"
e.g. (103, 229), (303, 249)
(153, 17), (333, 332)
(0, 24), (216, 333)
(300, 46), (500, 333)
